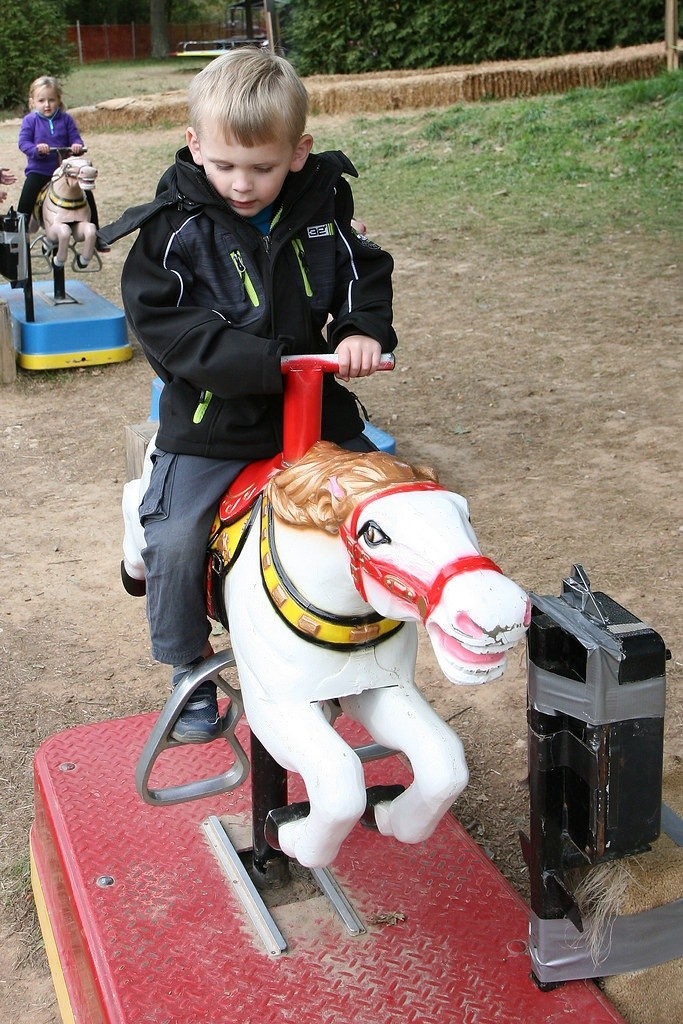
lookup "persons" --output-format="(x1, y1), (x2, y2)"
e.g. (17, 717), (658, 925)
(95, 46), (398, 744)
(17, 74), (111, 252)
(0, 167), (17, 204)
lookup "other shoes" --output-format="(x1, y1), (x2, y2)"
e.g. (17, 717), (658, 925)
(94, 238), (111, 252)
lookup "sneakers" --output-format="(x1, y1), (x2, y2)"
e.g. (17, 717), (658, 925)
(169, 656), (221, 742)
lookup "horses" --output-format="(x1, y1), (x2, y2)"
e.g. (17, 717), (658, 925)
(120, 429), (532, 869)
(28, 155), (99, 273)
